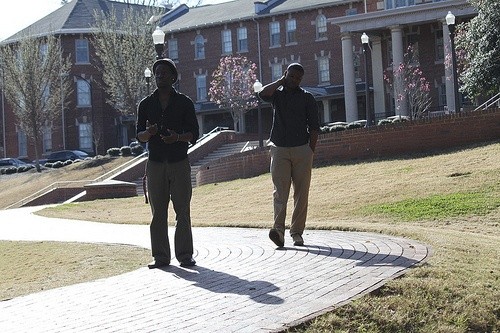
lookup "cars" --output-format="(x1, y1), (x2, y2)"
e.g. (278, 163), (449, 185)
(32, 150), (88, 164)
(0, 158), (26, 166)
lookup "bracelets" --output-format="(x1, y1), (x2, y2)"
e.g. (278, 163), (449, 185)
(176, 134), (180, 142)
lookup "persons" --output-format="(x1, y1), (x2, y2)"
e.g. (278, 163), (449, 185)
(259, 63), (320, 246)
(137, 58), (200, 269)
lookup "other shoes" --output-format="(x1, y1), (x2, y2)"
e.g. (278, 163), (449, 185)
(182, 258), (195, 266)
(293, 234), (304, 246)
(147, 260), (170, 267)
(269, 230), (284, 247)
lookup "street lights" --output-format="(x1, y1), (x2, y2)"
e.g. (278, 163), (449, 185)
(361, 33), (372, 125)
(445, 10), (460, 111)
(254, 80), (263, 146)
(144, 67), (152, 95)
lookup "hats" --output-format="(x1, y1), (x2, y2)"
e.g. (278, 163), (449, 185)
(153, 58), (178, 84)
